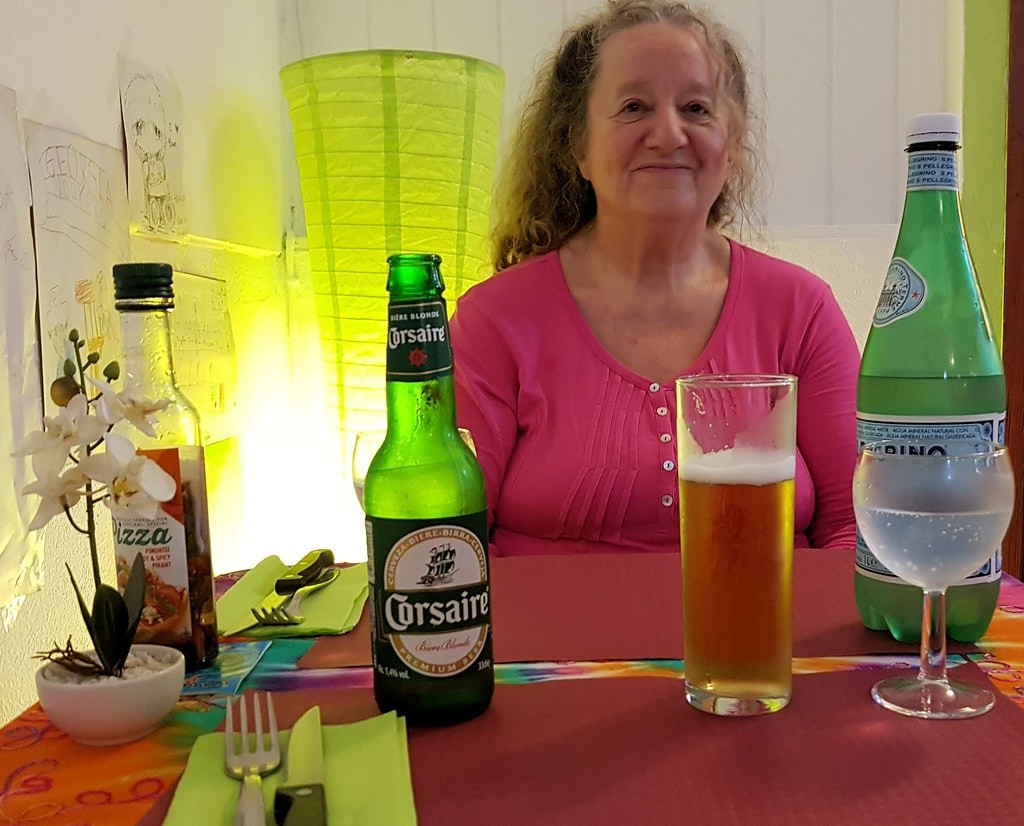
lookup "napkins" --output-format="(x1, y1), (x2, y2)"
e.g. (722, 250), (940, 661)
(161, 710), (418, 826)
(215, 555), (370, 639)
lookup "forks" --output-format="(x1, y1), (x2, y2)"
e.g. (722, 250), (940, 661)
(225, 692), (280, 826)
(251, 567), (340, 625)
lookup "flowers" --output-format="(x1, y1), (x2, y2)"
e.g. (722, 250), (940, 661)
(12, 328), (178, 676)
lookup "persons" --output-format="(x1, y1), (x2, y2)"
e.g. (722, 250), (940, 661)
(448, 0), (865, 555)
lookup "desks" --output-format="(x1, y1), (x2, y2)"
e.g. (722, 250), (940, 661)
(0, 541), (1024, 826)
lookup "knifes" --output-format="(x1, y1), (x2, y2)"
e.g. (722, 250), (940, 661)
(222, 549), (335, 637)
(275, 705), (327, 826)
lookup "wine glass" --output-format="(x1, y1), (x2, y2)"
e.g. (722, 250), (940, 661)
(853, 441), (1014, 717)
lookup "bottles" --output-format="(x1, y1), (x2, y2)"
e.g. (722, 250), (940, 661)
(362, 254), (495, 725)
(106, 264), (219, 670)
(854, 115), (1006, 640)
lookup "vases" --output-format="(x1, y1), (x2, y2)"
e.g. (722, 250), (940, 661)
(35, 643), (186, 746)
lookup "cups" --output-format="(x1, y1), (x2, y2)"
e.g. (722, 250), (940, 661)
(353, 429), (478, 511)
(677, 373), (798, 715)
